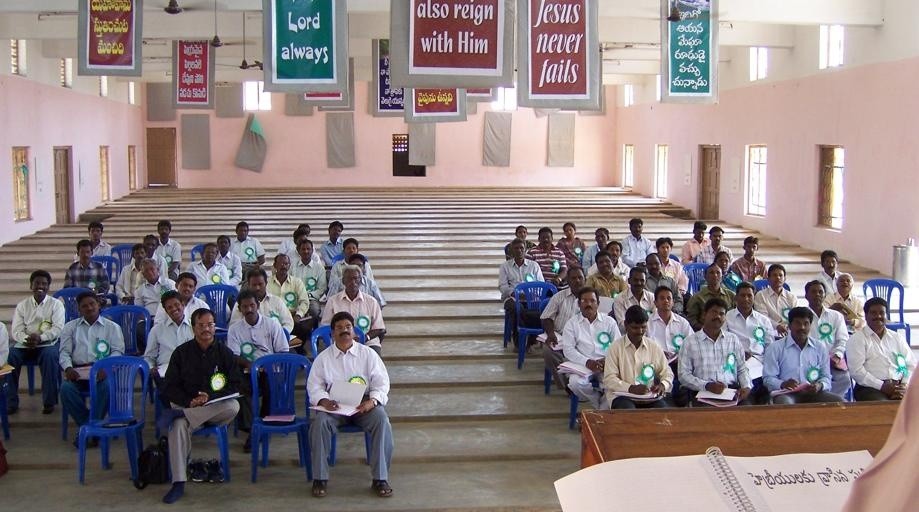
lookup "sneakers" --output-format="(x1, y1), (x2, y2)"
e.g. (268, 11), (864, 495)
(189, 458), (224, 482)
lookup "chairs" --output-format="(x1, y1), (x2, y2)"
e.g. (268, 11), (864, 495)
(504, 237), (914, 427)
(249, 353), (313, 487)
(2, 239), (375, 467)
(77, 354), (153, 489)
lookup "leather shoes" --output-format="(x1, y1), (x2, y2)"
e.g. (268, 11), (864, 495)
(41, 405), (54, 413)
(244, 433), (262, 451)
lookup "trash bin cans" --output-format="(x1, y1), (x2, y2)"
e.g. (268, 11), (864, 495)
(894, 245), (919, 288)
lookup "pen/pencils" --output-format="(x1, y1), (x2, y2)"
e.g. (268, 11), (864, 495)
(710, 379), (716, 383)
(637, 379), (644, 384)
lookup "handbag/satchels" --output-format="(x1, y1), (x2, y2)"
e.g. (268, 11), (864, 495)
(138, 444), (169, 484)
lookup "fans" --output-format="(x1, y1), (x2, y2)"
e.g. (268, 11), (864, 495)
(214, 10), (263, 72)
(143, 0), (229, 15)
(196, 1), (256, 50)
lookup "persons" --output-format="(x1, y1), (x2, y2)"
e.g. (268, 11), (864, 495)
(502, 216), (849, 410)
(822, 273), (866, 335)
(7, 269), (65, 416)
(306, 312), (394, 497)
(850, 373), (918, 511)
(58, 219), (387, 452)
(159, 308), (243, 506)
(845, 297), (916, 402)
(0, 321), (9, 474)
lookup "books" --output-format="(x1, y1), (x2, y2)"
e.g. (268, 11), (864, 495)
(0, 364), (15, 376)
(553, 446), (879, 512)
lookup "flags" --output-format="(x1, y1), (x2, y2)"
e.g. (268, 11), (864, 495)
(262, 0), (348, 94)
(516, 0), (601, 111)
(76, 1), (144, 79)
(659, 1), (721, 106)
(170, 38), (217, 110)
(386, 1), (517, 89)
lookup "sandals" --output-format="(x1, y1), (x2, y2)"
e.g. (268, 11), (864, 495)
(312, 479), (328, 497)
(372, 479), (393, 497)
